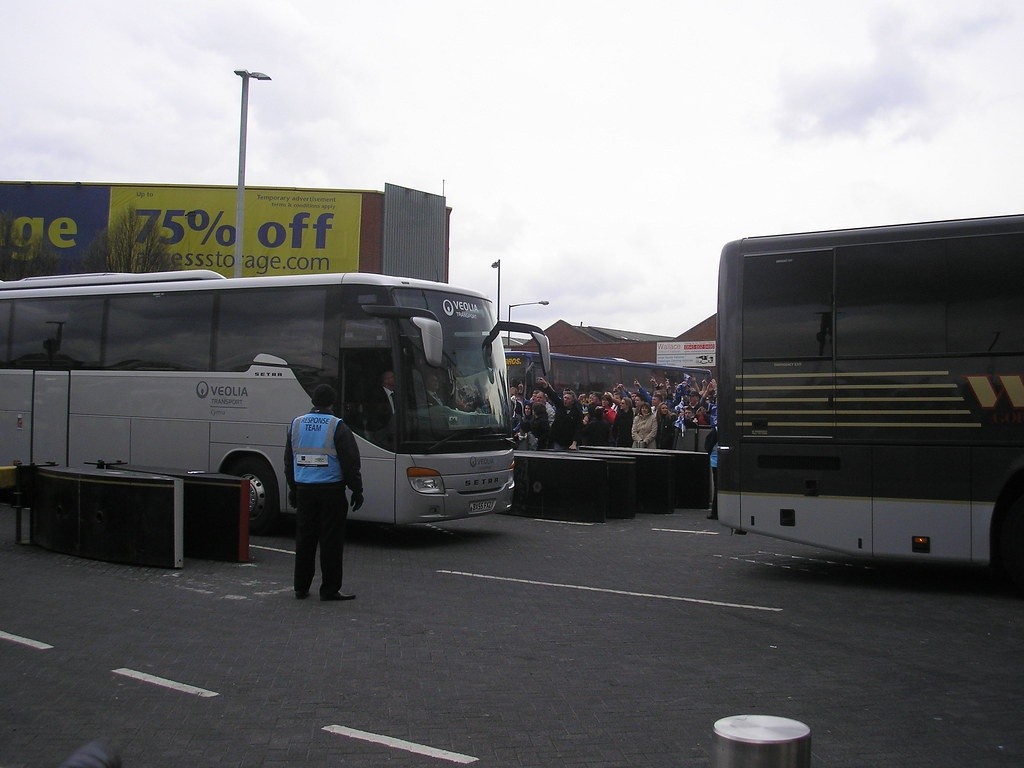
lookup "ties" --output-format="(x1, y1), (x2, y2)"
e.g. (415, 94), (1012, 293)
(390, 393), (396, 411)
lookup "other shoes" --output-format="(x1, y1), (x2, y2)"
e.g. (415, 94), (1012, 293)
(707, 514), (718, 520)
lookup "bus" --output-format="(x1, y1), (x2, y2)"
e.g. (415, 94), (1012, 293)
(714, 213), (1023, 602)
(0, 270), (551, 540)
(503, 350), (712, 402)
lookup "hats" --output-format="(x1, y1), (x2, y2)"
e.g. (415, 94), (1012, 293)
(312, 384), (336, 407)
(688, 391), (700, 397)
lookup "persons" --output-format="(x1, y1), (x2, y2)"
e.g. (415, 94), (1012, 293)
(415, 376), (446, 407)
(284, 384), (364, 601)
(705, 424), (719, 519)
(455, 375), (719, 451)
(365, 371), (399, 433)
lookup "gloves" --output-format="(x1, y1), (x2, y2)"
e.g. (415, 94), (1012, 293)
(350, 488), (364, 511)
(288, 489), (297, 508)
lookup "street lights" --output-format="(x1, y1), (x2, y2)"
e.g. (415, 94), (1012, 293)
(231, 66), (273, 277)
(507, 299), (550, 345)
(492, 258), (501, 325)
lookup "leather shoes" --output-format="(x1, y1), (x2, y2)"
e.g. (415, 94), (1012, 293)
(295, 594), (307, 599)
(320, 591), (356, 601)
